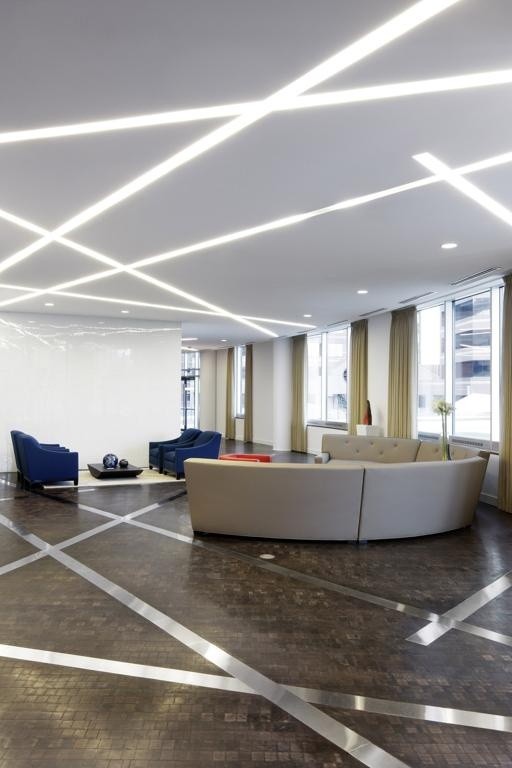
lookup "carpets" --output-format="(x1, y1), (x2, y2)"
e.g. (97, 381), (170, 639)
(41, 466), (186, 490)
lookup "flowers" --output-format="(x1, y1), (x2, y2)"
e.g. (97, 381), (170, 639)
(432, 398), (456, 461)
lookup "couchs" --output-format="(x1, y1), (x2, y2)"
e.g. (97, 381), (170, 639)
(183, 433), (491, 544)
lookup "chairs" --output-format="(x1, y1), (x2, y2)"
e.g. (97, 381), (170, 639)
(148, 427), (222, 480)
(10, 429), (79, 490)
